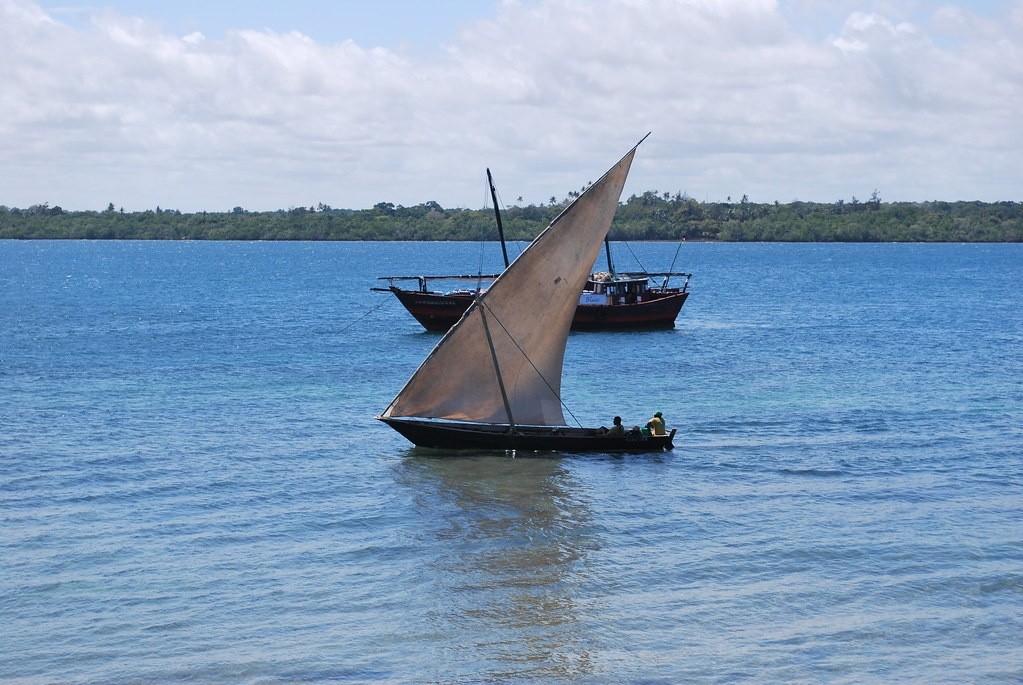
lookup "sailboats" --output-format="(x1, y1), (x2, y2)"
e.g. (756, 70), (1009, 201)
(375, 131), (677, 451)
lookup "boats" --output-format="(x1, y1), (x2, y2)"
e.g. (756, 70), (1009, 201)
(370, 167), (693, 332)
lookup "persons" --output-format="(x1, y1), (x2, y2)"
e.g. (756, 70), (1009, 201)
(644, 411), (665, 436)
(596, 416), (624, 435)
